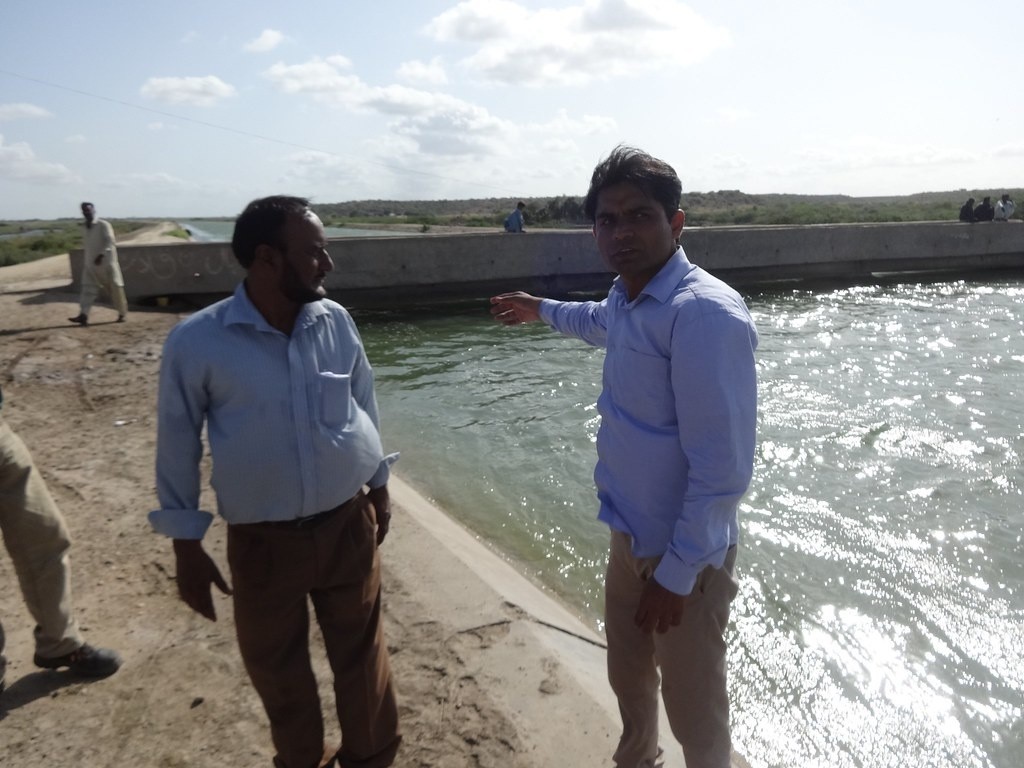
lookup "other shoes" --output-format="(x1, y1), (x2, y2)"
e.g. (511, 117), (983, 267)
(33, 639), (122, 676)
(118, 315), (127, 322)
(67, 313), (88, 324)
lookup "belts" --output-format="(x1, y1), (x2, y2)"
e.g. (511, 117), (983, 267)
(291, 482), (371, 529)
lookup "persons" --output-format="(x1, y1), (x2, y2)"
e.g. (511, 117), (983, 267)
(146, 195), (400, 768)
(994, 192), (1015, 221)
(959, 198), (977, 222)
(67, 202), (128, 325)
(0, 385), (123, 716)
(974, 196), (994, 221)
(491, 141), (760, 768)
(510, 201), (526, 233)
